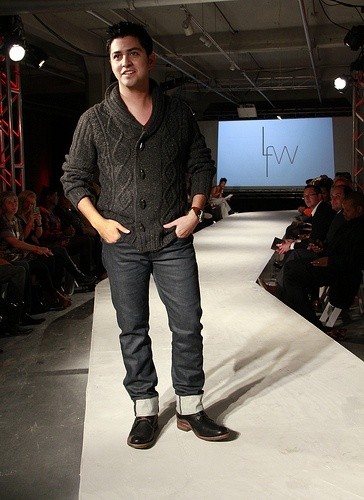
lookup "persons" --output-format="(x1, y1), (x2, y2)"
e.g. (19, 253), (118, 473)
(274, 172), (364, 336)
(0, 184), (96, 349)
(210, 178), (234, 219)
(60, 22), (231, 448)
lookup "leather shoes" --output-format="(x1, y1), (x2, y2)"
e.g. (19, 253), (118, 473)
(177, 410), (231, 441)
(127, 414), (158, 449)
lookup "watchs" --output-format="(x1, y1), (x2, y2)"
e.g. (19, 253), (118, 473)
(190, 207), (204, 223)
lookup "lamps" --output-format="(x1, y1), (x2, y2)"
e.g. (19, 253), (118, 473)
(200, 34), (212, 49)
(344, 25), (364, 51)
(334, 74), (354, 94)
(183, 15), (194, 36)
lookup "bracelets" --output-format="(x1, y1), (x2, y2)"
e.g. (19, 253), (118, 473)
(36, 223), (42, 227)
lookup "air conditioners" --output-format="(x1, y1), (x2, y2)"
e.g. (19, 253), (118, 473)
(28, 45), (48, 69)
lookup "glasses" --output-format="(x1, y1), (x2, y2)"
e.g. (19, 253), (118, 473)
(301, 193), (319, 199)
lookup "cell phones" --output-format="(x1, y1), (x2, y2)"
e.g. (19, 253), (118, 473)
(33, 206), (39, 219)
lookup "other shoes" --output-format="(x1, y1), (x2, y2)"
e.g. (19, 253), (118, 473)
(76, 272), (97, 287)
(0, 319), (33, 336)
(14, 312), (45, 326)
